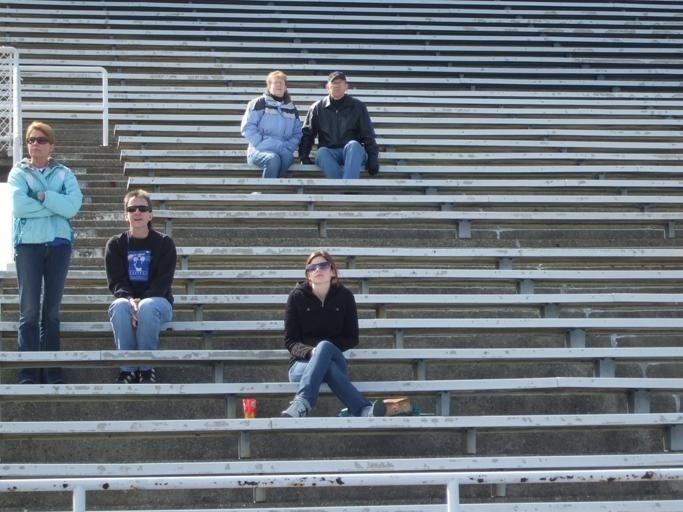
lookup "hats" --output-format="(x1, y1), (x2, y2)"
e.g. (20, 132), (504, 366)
(328, 71), (346, 83)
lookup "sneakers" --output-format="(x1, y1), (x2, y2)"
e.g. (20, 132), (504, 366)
(138, 368), (156, 383)
(368, 398), (387, 416)
(19, 377), (65, 383)
(114, 370), (138, 383)
(281, 400), (308, 417)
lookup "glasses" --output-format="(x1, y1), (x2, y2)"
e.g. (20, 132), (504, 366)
(126, 205), (151, 212)
(25, 136), (51, 145)
(306, 260), (333, 272)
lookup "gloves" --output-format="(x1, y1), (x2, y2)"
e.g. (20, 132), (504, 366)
(301, 159), (314, 165)
(365, 161), (379, 174)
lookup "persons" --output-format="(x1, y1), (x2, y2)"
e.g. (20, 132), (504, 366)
(296, 71), (380, 194)
(279, 250), (388, 418)
(238, 71), (303, 195)
(103, 189), (177, 384)
(5, 121), (83, 385)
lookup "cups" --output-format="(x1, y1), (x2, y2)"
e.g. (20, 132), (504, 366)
(241, 398), (256, 418)
